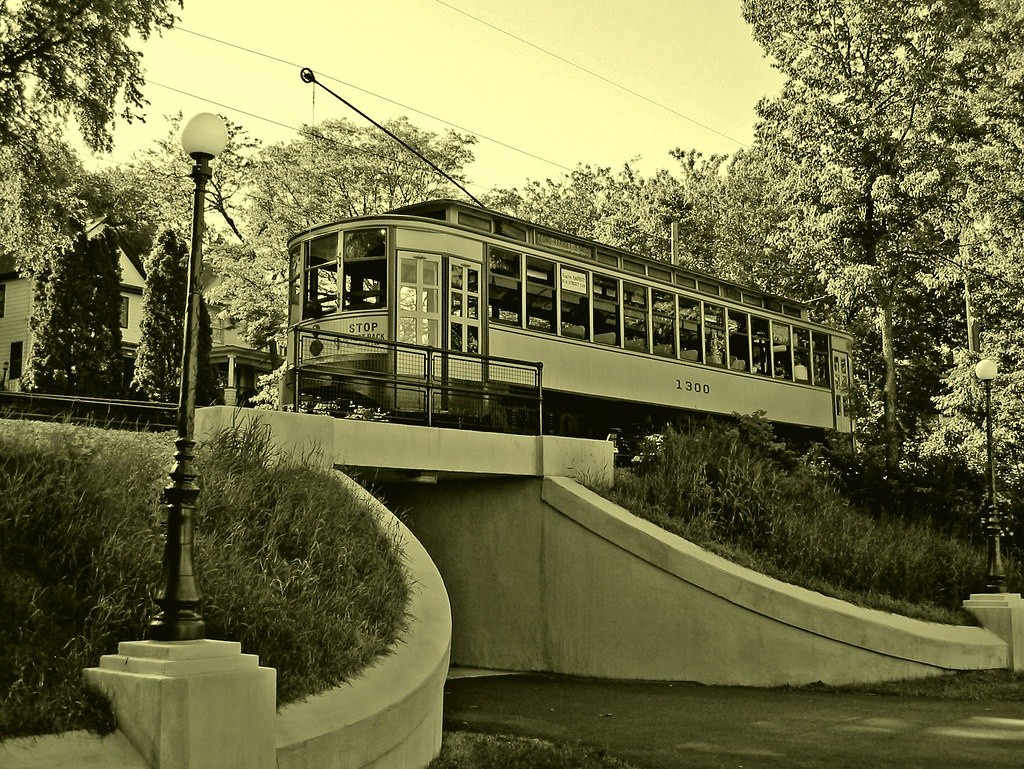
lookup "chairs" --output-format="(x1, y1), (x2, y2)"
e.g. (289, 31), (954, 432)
(562, 326), (745, 371)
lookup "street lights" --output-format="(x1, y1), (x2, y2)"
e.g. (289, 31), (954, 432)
(976, 359), (1008, 593)
(145, 114), (229, 641)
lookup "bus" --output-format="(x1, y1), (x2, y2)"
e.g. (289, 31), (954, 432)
(285, 68), (855, 454)
